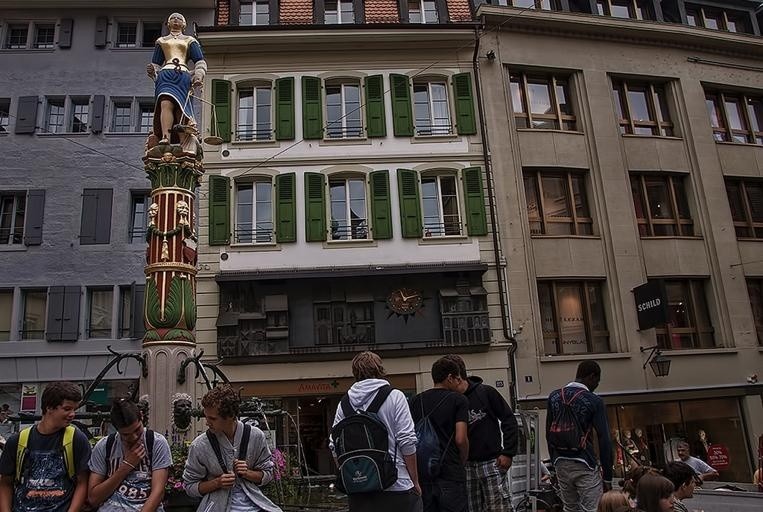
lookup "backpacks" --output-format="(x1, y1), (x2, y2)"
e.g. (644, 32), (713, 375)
(414, 391), (458, 484)
(548, 387), (590, 454)
(329, 384), (400, 496)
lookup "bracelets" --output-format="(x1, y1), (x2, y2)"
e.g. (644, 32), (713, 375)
(121, 458), (135, 472)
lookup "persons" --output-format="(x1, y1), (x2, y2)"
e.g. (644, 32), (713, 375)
(674, 439), (720, 490)
(86, 396), (174, 511)
(407, 357), (472, 512)
(662, 460), (696, 511)
(0, 379), (91, 511)
(636, 470), (676, 512)
(539, 460), (552, 485)
(599, 487), (633, 511)
(621, 465), (657, 498)
(180, 383), (285, 511)
(327, 349), (424, 512)
(621, 428), (639, 470)
(439, 352), (520, 512)
(177, 201), (188, 219)
(614, 429), (624, 465)
(633, 426), (651, 466)
(544, 359), (614, 511)
(146, 203), (158, 221)
(0, 402), (13, 421)
(692, 428), (711, 462)
(144, 8), (207, 146)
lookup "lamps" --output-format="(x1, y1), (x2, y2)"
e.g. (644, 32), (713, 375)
(639, 344), (670, 379)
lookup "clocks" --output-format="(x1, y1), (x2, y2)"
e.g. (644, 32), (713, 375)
(373, 281), (433, 324)
